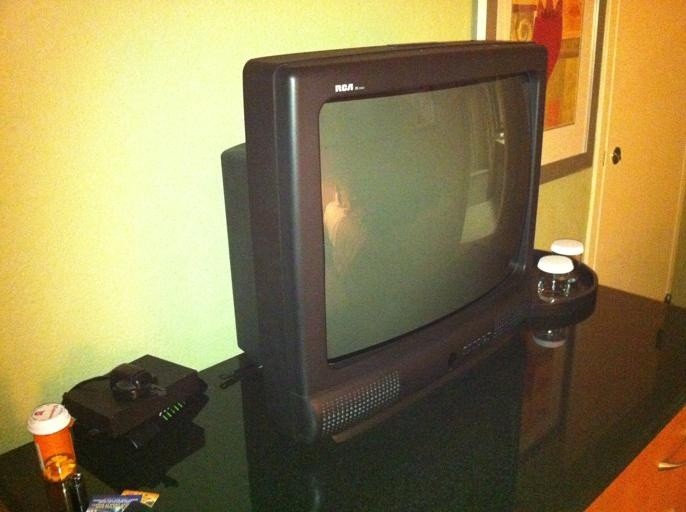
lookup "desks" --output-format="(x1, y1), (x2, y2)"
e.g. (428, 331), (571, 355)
(0, 282), (686, 512)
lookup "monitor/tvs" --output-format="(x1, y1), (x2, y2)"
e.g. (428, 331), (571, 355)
(221, 40), (548, 443)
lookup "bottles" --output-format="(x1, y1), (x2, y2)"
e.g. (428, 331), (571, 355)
(532, 320), (569, 349)
(536, 237), (586, 303)
(26, 402), (77, 485)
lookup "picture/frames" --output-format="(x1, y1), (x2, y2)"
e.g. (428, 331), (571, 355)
(487, 1), (608, 186)
